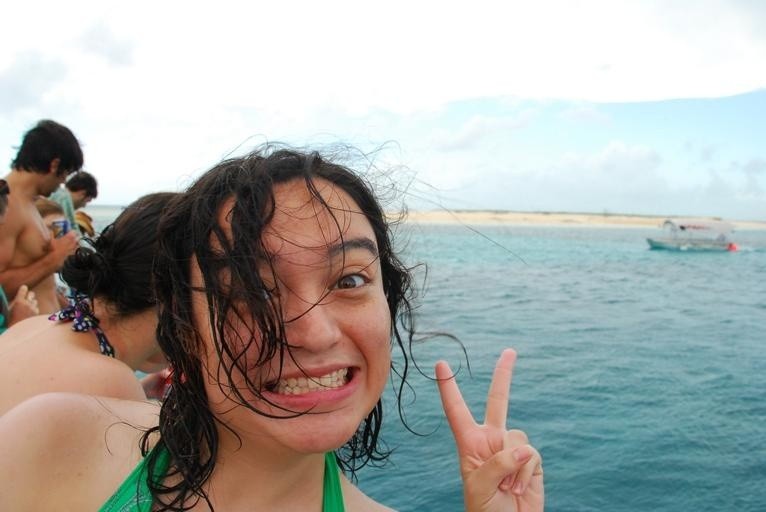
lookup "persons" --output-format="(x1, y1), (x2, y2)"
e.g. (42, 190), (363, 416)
(1, 118), (98, 333)
(2, 149), (545, 511)
(2, 192), (183, 414)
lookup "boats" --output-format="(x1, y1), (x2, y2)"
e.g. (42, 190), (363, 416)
(646, 221), (736, 253)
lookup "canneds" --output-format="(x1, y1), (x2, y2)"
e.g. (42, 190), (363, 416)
(52, 220), (70, 239)
